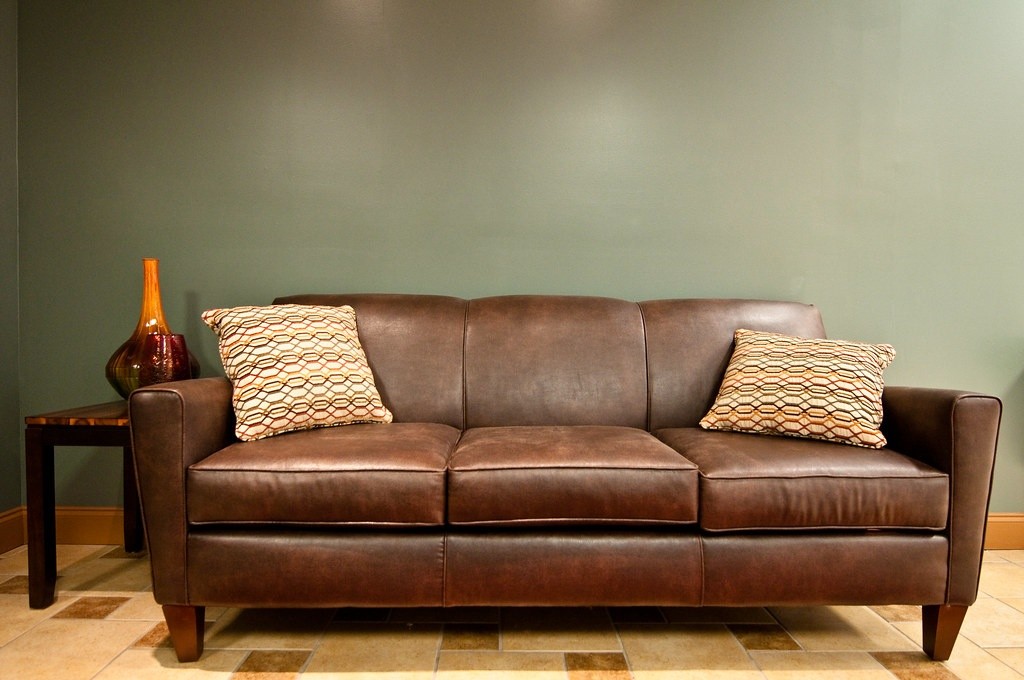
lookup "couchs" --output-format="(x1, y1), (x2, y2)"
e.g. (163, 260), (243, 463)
(125, 291), (1003, 663)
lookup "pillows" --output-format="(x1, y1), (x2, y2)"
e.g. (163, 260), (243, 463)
(198, 303), (394, 443)
(698, 328), (897, 450)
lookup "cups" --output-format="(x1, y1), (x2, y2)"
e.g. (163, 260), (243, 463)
(140, 334), (188, 389)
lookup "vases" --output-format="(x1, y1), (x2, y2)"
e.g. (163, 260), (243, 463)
(140, 332), (191, 389)
(105, 257), (200, 399)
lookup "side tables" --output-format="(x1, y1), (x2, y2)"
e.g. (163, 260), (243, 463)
(23, 399), (144, 609)
